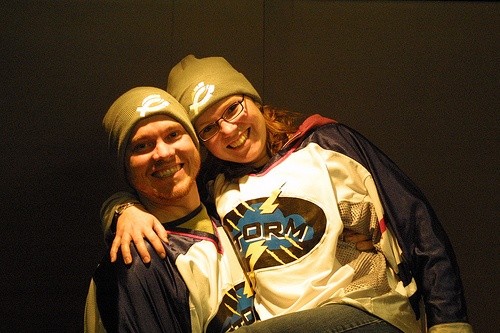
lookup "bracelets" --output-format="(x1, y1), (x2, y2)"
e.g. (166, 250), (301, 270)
(109, 202), (146, 238)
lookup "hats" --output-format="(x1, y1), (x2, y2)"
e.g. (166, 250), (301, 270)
(101, 85), (198, 182)
(167, 55), (261, 125)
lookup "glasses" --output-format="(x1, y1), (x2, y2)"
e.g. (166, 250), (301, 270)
(196, 95), (245, 142)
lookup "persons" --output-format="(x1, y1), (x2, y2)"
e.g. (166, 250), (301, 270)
(84, 85), (260, 333)
(97, 50), (473, 333)
